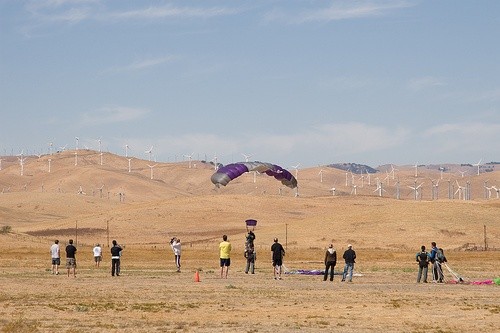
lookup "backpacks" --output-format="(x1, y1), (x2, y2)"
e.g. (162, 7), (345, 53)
(432, 249), (444, 262)
(420, 252), (427, 266)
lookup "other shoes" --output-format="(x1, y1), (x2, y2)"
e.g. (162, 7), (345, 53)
(342, 280), (345, 281)
(323, 280), (326, 281)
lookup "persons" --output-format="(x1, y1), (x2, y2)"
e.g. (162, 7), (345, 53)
(271, 237), (286, 280)
(93, 243), (102, 267)
(341, 244), (357, 282)
(110, 239), (123, 277)
(323, 244), (337, 281)
(416, 245), (431, 284)
(48, 239), (61, 275)
(429, 241), (446, 284)
(169, 237), (182, 272)
(244, 230), (256, 274)
(218, 234), (231, 279)
(65, 239), (77, 278)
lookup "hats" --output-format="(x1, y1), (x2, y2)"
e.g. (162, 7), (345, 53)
(348, 244), (352, 247)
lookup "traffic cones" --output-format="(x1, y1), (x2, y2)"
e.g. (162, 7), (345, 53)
(193, 270), (200, 282)
